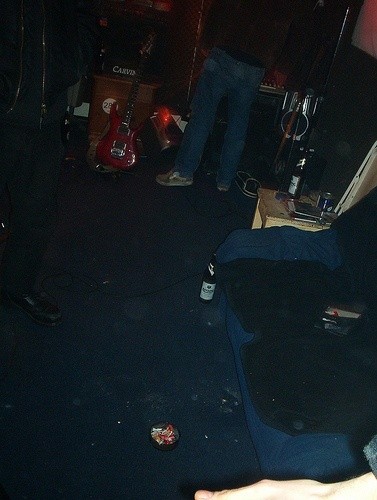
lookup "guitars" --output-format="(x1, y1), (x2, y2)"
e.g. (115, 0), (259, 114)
(96, 32), (158, 171)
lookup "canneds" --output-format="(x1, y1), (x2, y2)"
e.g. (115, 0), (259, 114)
(317, 192), (334, 212)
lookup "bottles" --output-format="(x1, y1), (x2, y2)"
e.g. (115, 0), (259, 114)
(95, 41), (106, 74)
(287, 150), (309, 199)
(199, 253), (218, 303)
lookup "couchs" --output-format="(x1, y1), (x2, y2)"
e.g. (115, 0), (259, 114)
(214, 189), (377, 484)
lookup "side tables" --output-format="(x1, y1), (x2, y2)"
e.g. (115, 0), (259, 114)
(249, 187), (331, 234)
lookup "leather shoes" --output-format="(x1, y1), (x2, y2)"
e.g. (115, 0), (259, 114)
(0, 288), (62, 326)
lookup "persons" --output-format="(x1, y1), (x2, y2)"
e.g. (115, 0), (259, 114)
(155, 0), (298, 192)
(0, 0), (80, 327)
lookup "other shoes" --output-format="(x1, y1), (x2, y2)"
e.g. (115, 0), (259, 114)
(217, 183), (229, 192)
(156, 170), (193, 186)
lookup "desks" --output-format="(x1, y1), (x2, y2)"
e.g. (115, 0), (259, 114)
(219, 90), (283, 167)
(90, 71), (162, 146)
